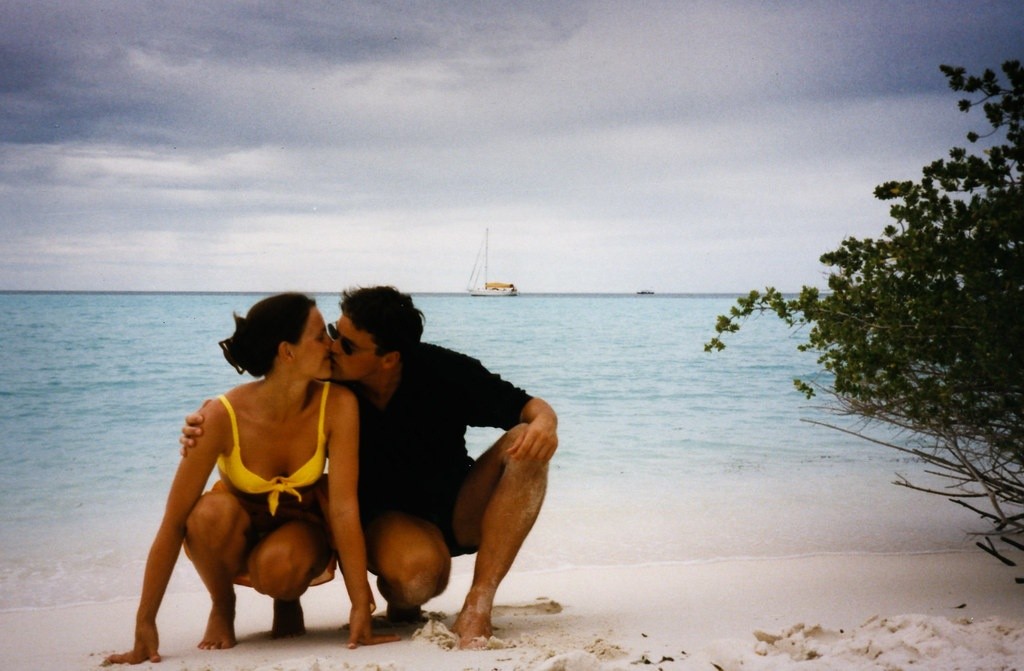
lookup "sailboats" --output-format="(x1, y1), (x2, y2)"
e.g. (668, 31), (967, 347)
(463, 226), (518, 297)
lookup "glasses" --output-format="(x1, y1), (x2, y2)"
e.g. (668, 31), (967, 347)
(328, 322), (380, 355)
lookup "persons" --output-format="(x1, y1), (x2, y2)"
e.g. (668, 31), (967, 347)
(179, 285), (560, 651)
(98, 293), (401, 666)
